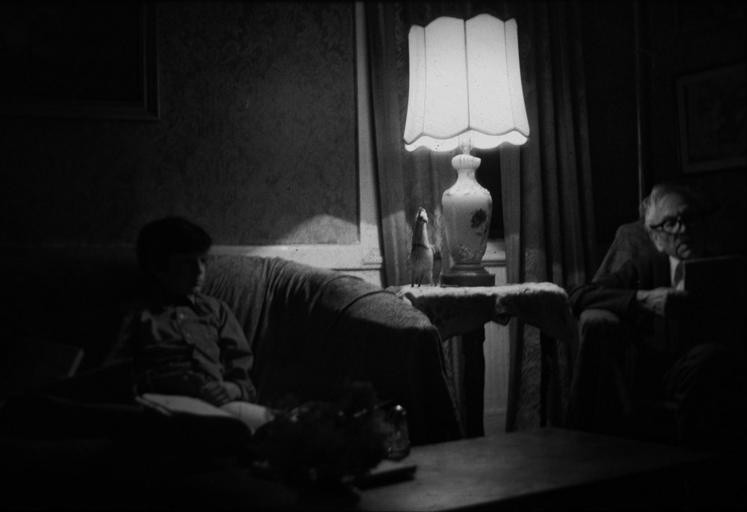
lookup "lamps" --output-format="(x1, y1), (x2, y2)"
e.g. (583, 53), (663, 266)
(402, 13), (533, 285)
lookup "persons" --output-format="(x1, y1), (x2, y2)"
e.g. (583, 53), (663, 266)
(108, 217), (257, 407)
(570, 186), (747, 342)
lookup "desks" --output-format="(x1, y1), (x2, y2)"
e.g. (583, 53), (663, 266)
(386, 282), (573, 439)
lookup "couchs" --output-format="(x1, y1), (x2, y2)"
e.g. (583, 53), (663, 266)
(1, 252), (441, 498)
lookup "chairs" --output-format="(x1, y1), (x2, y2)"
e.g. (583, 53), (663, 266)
(557, 219), (746, 441)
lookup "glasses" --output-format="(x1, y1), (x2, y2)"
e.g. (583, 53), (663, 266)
(649, 206), (697, 235)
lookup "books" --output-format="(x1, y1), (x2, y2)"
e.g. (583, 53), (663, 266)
(138, 394), (290, 436)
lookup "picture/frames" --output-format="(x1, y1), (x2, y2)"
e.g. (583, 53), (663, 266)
(670, 58), (747, 175)
(0, 1), (162, 123)
